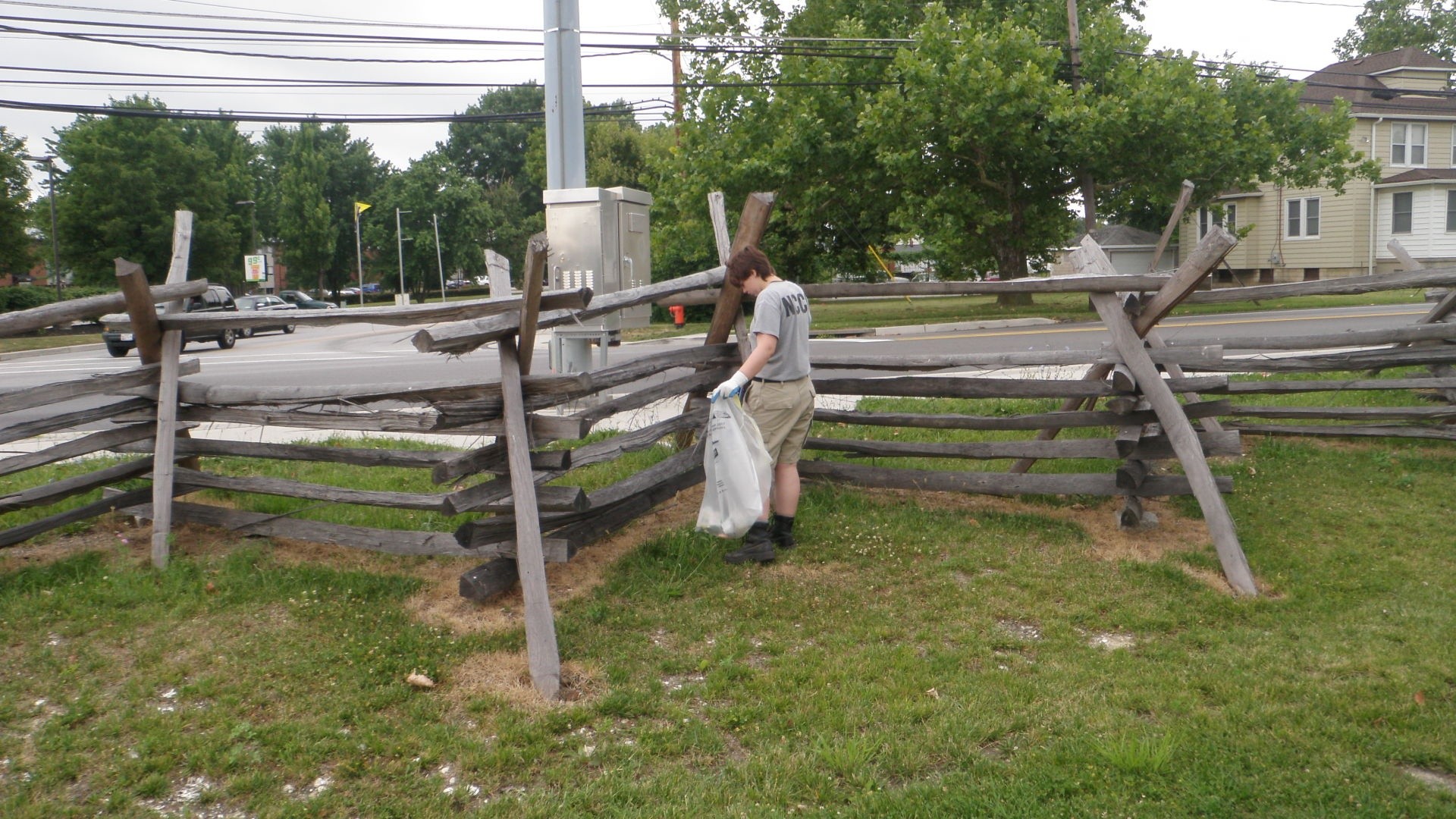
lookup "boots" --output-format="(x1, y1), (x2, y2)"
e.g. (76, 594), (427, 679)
(765, 513), (798, 551)
(724, 522), (775, 563)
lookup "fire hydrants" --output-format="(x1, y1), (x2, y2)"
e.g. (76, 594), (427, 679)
(669, 306), (685, 328)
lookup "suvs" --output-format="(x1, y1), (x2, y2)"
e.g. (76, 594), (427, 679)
(98, 282), (238, 358)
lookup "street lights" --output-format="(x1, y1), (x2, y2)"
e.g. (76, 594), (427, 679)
(22, 156), (61, 302)
(236, 200), (257, 255)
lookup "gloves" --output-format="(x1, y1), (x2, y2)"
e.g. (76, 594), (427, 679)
(710, 370), (748, 404)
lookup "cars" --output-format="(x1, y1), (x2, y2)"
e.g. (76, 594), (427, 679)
(232, 294), (297, 339)
(444, 275), (490, 290)
(307, 284), (380, 296)
(912, 268), (999, 283)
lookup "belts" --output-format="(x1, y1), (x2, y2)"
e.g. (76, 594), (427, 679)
(751, 376), (789, 383)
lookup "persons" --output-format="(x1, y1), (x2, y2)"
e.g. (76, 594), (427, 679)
(285, 295), (298, 302)
(188, 298), (199, 310)
(712, 245), (815, 564)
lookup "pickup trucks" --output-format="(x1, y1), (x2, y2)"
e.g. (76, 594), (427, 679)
(840, 269), (909, 282)
(280, 291), (338, 309)
